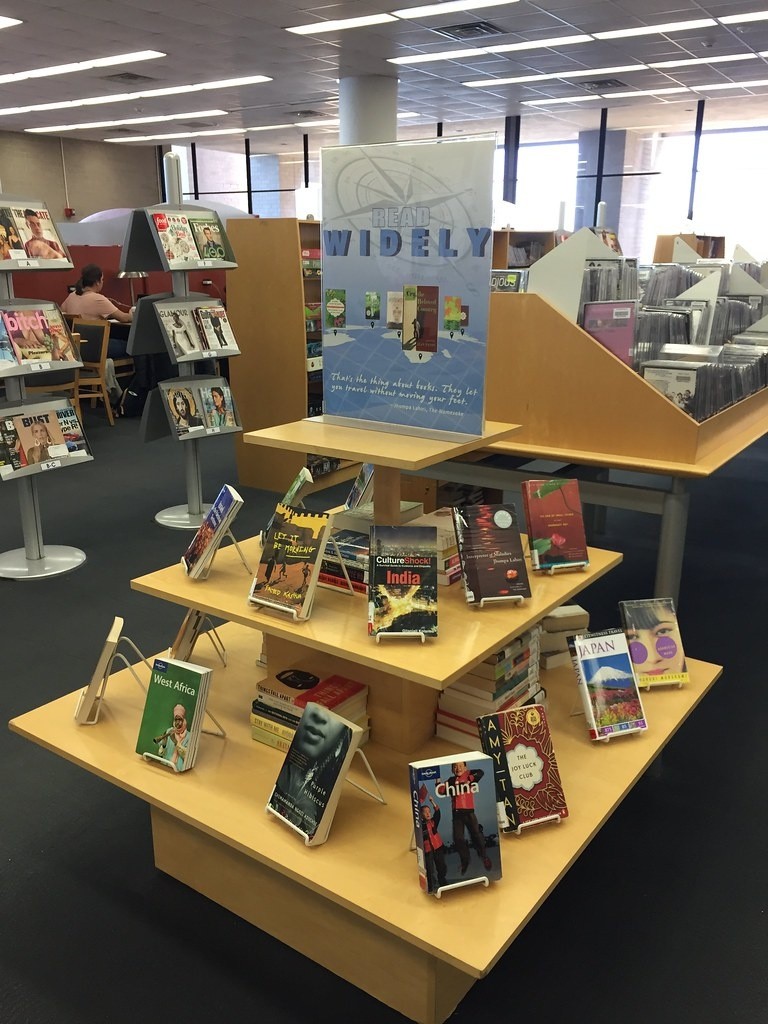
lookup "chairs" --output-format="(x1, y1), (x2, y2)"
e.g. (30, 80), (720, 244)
(22, 313), (87, 430)
(64, 315), (135, 410)
(69, 315), (117, 427)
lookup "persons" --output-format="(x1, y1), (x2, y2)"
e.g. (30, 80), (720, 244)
(59, 264), (135, 413)
(8, 227), (23, 249)
(24, 209), (65, 260)
(171, 313), (197, 354)
(209, 387), (234, 427)
(173, 391), (203, 430)
(620, 598), (687, 678)
(278, 702), (346, 835)
(435, 761), (492, 872)
(159, 704), (190, 768)
(0, 235), (11, 259)
(27, 421), (58, 465)
(203, 227), (225, 260)
(210, 312), (228, 348)
(14, 432), (28, 468)
(418, 797), (448, 892)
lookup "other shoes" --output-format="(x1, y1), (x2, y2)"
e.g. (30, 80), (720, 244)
(461, 864), (467, 875)
(428, 883), (435, 892)
(484, 858), (492, 870)
(438, 877), (447, 885)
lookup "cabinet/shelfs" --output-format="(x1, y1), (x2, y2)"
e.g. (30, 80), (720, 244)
(0, 197), (768, 1024)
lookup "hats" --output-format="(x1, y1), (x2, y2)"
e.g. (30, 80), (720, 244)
(174, 704), (185, 719)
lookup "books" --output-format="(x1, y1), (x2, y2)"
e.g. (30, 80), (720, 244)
(164, 386), (237, 436)
(0, 207), (91, 477)
(300, 236), (767, 477)
(160, 306), (239, 358)
(153, 213), (230, 264)
(79, 463), (690, 896)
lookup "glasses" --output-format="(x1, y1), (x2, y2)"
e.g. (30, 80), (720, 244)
(175, 718), (181, 721)
